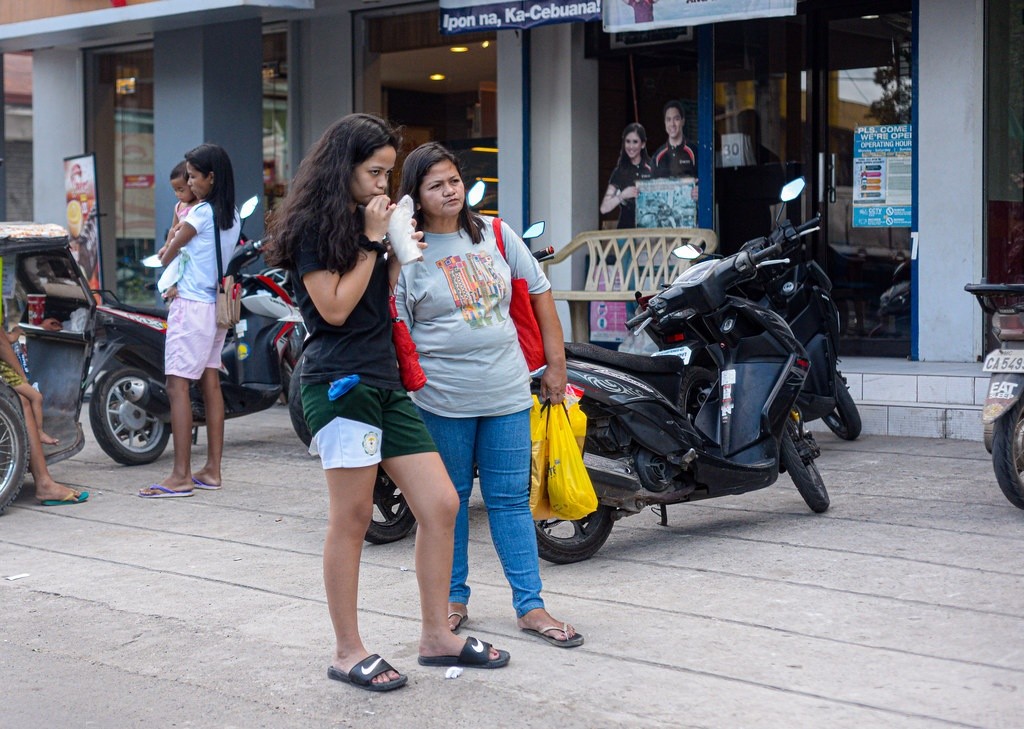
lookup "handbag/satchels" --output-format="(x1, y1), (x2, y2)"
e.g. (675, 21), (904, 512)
(389, 295), (427, 392)
(215, 275), (242, 330)
(530, 393), (599, 520)
(492, 216), (548, 372)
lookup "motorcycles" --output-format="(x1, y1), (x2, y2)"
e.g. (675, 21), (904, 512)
(529, 174), (861, 567)
(83, 193), (311, 463)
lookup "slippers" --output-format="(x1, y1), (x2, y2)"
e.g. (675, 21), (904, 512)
(417, 636), (510, 668)
(42, 490), (89, 506)
(522, 621), (584, 647)
(327, 654), (408, 691)
(138, 483), (194, 498)
(191, 476), (222, 491)
(448, 612), (468, 635)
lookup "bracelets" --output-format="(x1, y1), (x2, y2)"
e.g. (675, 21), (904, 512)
(358, 234), (387, 257)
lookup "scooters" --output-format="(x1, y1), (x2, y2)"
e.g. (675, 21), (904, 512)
(965, 282), (1024, 510)
(287, 183), (554, 543)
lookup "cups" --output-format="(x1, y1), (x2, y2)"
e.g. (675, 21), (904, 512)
(387, 203), (423, 265)
(27, 294), (47, 326)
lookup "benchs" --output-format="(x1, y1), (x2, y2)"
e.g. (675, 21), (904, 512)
(543, 227), (718, 343)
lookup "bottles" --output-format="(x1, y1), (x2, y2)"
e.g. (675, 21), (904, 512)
(11, 341), (32, 380)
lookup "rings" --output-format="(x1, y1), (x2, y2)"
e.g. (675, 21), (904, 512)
(562, 394), (565, 397)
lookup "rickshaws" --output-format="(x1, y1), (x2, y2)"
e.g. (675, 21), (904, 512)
(0, 222), (98, 517)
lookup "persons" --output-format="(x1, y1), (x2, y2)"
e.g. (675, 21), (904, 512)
(261, 111), (511, 692)
(598, 101), (700, 228)
(138, 144), (242, 499)
(717, 110), (787, 251)
(0, 316), (88, 505)
(383, 142), (584, 647)
